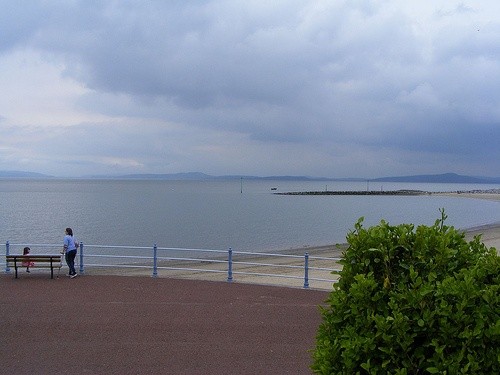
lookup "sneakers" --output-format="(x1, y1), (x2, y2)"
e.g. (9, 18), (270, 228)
(66, 273), (77, 279)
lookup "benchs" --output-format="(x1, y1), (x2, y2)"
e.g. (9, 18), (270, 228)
(6, 254), (63, 278)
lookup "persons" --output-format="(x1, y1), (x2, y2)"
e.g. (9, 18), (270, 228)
(21, 247), (34, 272)
(61, 228), (79, 278)
